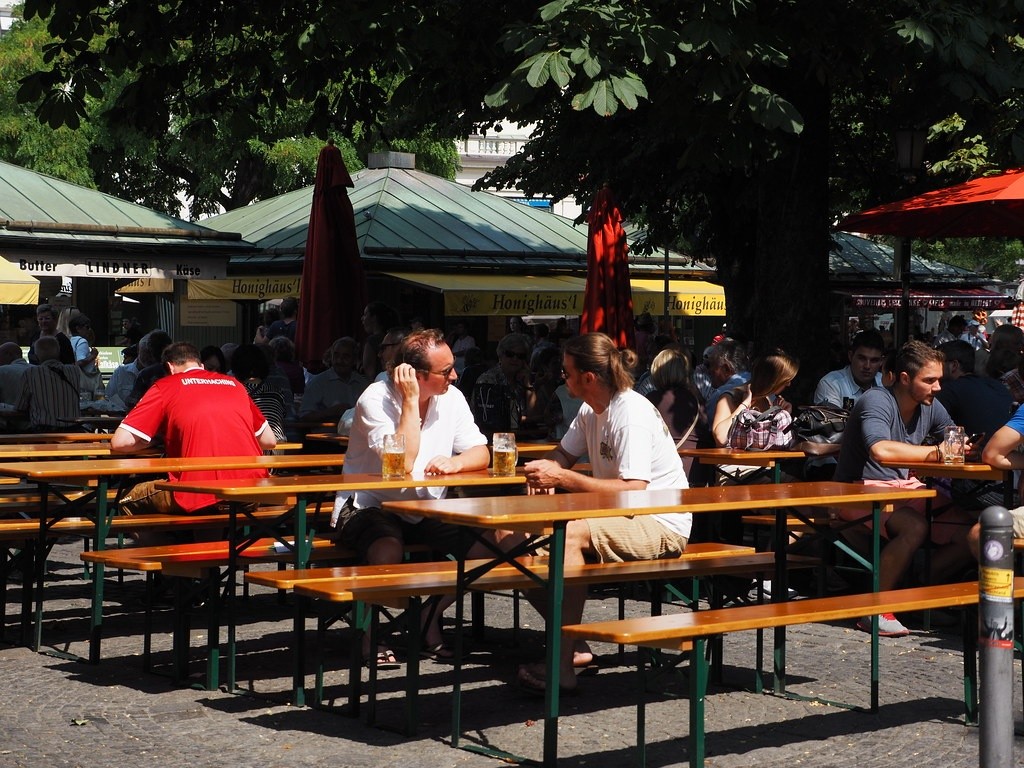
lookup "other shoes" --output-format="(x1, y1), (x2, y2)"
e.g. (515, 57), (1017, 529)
(749, 578), (797, 603)
(146, 572), (207, 611)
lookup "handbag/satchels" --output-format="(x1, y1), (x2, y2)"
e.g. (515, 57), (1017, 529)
(728, 409), (793, 451)
(786, 395), (856, 457)
(74, 338), (105, 391)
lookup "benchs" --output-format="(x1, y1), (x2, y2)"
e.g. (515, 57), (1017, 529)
(0, 479), (868, 768)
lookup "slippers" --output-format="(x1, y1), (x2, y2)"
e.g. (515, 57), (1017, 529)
(396, 637), (466, 661)
(360, 638), (403, 667)
(518, 657), (574, 694)
(572, 660), (599, 677)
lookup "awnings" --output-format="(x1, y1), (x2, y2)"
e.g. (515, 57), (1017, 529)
(383, 273), (726, 316)
(116, 277), (299, 300)
(0, 256), (39, 305)
(832, 288), (1015, 310)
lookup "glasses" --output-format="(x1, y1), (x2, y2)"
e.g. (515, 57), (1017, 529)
(418, 356), (457, 379)
(503, 349), (527, 360)
(380, 342), (400, 352)
(560, 366), (591, 379)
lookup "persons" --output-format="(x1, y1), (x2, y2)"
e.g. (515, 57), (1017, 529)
(330, 328), (490, 668)
(106, 297), (1024, 637)
(523, 332), (693, 691)
(111, 341), (277, 610)
(0, 304), (106, 434)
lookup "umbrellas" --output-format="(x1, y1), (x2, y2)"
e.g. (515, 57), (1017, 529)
(297, 138), (359, 373)
(837, 166), (1024, 240)
(578, 183), (637, 354)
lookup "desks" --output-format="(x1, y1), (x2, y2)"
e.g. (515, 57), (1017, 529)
(382, 483), (938, 768)
(559, 576), (1024, 768)
(0, 431), (1024, 508)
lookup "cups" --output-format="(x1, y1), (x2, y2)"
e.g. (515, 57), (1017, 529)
(943, 426), (965, 468)
(492, 432), (518, 477)
(382, 434), (407, 482)
(94, 389), (110, 403)
(79, 391), (91, 403)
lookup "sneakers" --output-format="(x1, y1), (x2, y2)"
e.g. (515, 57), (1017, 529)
(860, 612), (908, 638)
